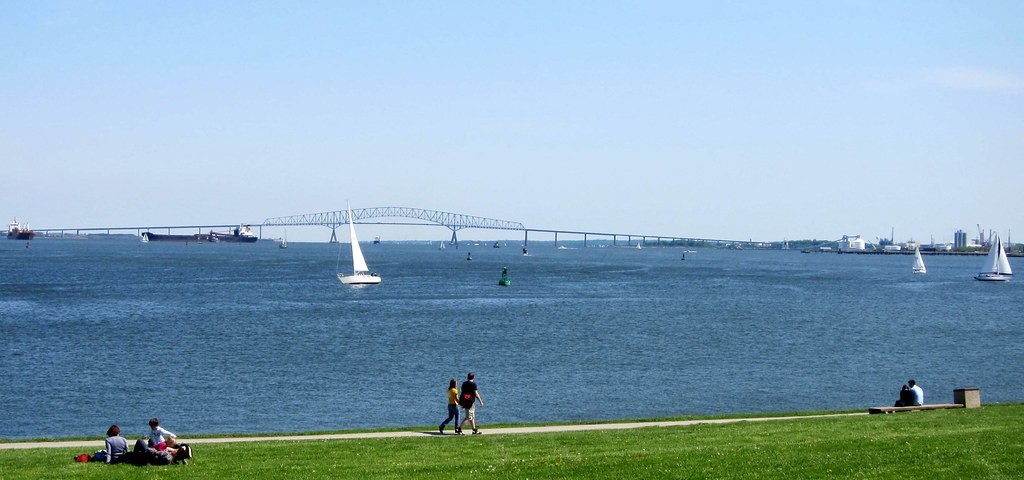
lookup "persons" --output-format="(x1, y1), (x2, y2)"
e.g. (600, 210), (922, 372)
(105, 425), (129, 464)
(439, 378), (460, 434)
(458, 372), (484, 434)
(895, 385), (916, 412)
(908, 380), (923, 405)
(151, 436), (178, 452)
(142, 418), (176, 445)
(502, 266), (508, 280)
(133, 439), (179, 465)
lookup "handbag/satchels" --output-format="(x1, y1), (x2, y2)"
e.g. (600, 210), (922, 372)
(458, 392), (475, 409)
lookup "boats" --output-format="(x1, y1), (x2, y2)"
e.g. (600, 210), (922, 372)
(374, 236), (381, 244)
(493, 241), (501, 249)
(466, 252), (473, 260)
(497, 267), (512, 286)
(279, 238), (288, 249)
(210, 220), (258, 243)
(6, 217), (37, 240)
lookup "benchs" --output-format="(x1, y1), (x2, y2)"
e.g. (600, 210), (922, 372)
(868, 404), (964, 414)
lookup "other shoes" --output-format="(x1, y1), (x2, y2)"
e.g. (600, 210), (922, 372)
(457, 429), (465, 435)
(438, 426), (444, 434)
(472, 429), (484, 434)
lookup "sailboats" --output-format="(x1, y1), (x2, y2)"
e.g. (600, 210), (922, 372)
(912, 245), (928, 274)
(139, 232), (150, 243)
(438, 241), (446, 251)
(974, 231), (1016, 281)
(336, 197), (381, 286)
(635, 242), (642, 250)
